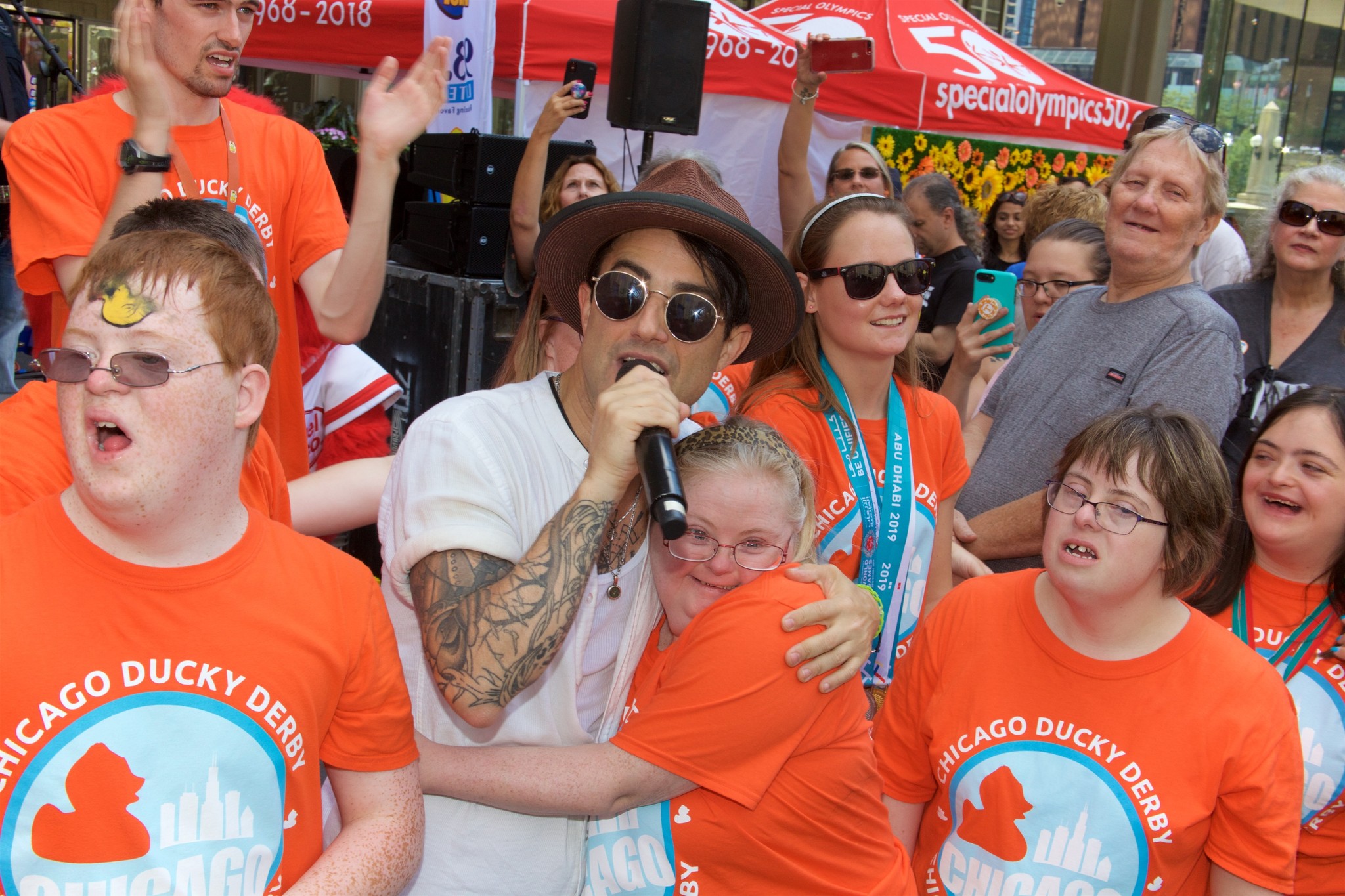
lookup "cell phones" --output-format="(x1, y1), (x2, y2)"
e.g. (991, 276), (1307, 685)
(971, 269), (1017, 358)
(561, 58), (597, 120)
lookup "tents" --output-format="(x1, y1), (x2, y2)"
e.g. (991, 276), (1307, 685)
(236, 0), (1161, 250)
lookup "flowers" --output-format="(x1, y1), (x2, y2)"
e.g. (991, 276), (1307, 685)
(307, 96), (412, 164)
(876, 132), (1116, 223)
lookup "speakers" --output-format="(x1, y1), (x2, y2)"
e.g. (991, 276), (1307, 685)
(406, 126), (598, 279)
(606, 0), (712, 136)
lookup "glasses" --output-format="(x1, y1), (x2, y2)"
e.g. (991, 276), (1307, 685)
(662, 529), (793, 572)
(996, 191), (1028, 204)
(806, 257), (937, 301)
(28, 347), (229, 389)
(1043, 479), (1170, 535)
(829, 167), (884, 181)
(1141, 112), (1227, 175)
(1278, 200), (1345, 236)
(588, 269), (728, 345)
(1016, 278), (1104, 299)
(1235, 365), (1277, 418)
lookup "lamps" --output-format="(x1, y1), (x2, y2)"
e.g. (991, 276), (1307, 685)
(1270, 136), (1284, 161)
(1250, 134), (1262, 159)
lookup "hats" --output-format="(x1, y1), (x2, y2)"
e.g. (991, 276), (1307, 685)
(530, 155), (805, 368)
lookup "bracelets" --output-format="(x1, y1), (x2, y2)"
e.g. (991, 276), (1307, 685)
(856, 584), (885, 640)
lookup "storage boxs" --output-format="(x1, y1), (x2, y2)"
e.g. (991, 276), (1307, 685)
(361, 264), (525, 454)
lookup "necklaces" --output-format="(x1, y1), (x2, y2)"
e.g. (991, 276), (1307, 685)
(557, 372), (643, 542)
(554, 372), (643, 600)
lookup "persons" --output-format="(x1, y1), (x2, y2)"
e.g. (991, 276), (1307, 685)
(0, 7), (1345, 896)
(0, 0), (453, 483)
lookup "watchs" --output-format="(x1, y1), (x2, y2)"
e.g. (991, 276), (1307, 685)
(115, 140), (174, 175)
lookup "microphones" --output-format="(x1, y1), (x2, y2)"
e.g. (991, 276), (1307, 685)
(615, 359), (689, 539)
